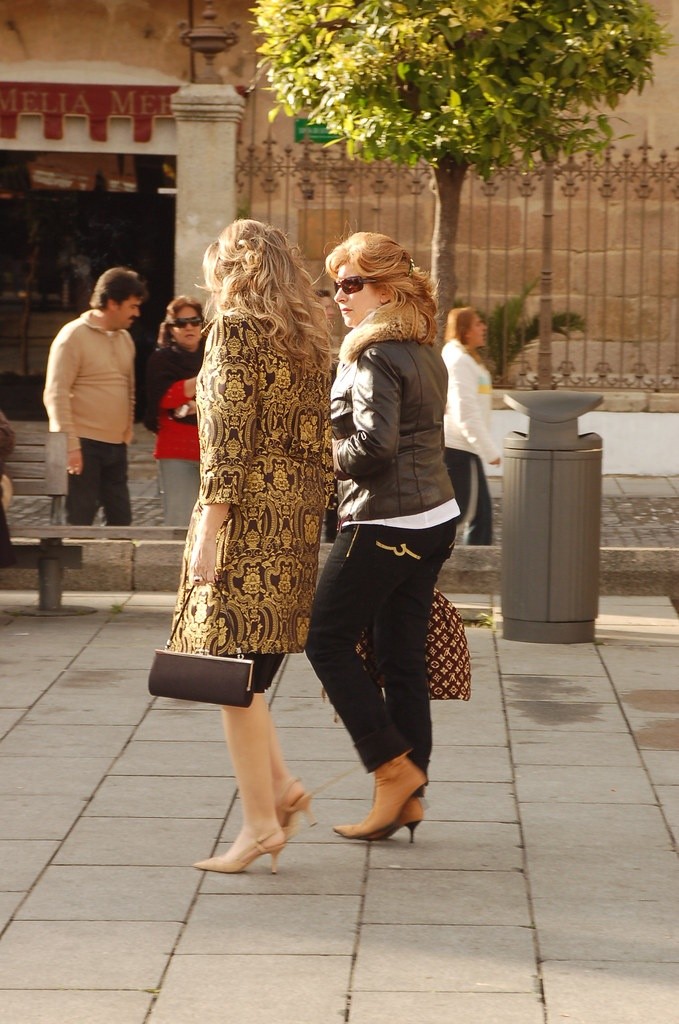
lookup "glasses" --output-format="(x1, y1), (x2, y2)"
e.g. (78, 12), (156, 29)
(333, 276), (379, 295)
(168, 316), (203, 329)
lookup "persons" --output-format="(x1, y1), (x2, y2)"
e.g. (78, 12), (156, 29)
(143, 296), (207, 527)
(166, 219), (333, 874)
(0, 412), (16, 571)
(311, 289), (338, 543)
(43, 267), (144, 541)
(305, 231), (459, 843)
(441, 307), (500, 546)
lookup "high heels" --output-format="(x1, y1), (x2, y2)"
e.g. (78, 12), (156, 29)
(191, 826), (287, 873)
(358, 771), (424, 843)
(274, 776), (318, 842)
(331, 756), (430, 839)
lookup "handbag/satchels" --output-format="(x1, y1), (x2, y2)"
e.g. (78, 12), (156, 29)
(353, 586), (471, 701)
(149, 576), (255, 708)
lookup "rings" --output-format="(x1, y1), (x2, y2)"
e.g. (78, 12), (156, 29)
(75, 464), (78, 469)
(195, 578), (202, 582)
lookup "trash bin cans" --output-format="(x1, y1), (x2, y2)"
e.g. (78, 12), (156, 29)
(501, 390), (603, 644)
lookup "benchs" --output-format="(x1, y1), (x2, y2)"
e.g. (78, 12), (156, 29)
(0, 433), (96, 616)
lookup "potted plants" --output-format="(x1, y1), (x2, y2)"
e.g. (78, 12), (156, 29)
(454, 275), (584, 392)
